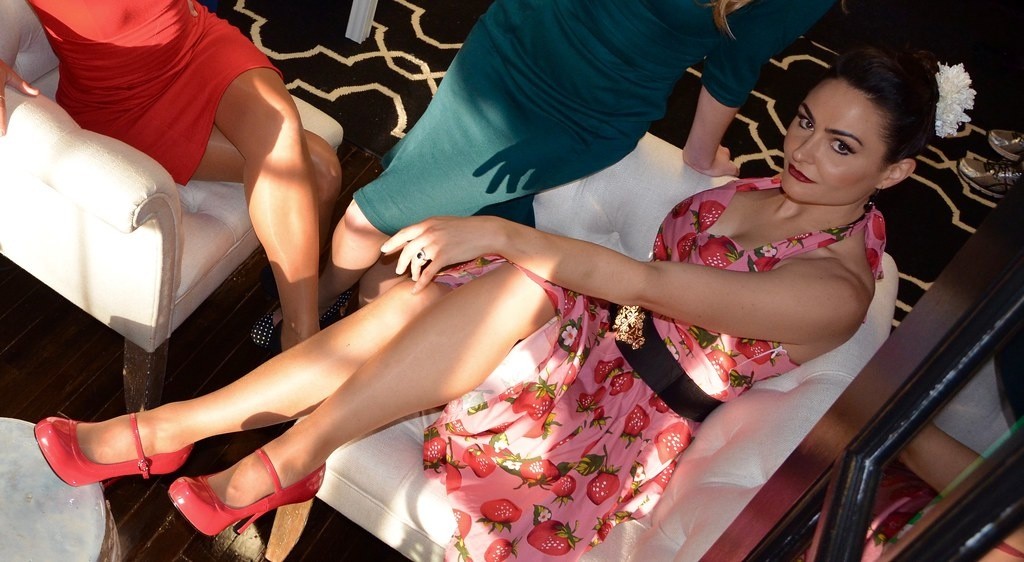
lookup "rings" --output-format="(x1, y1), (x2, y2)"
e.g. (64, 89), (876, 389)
(416, 250), (428, 262)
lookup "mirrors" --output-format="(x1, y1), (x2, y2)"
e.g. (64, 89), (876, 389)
(816, 261), (1024, 562)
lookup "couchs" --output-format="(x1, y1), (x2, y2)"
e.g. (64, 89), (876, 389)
(316, 127), (897, 561)
(0, 0), (344, 416)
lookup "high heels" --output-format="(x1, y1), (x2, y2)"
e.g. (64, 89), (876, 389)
(32, 411), (195, 490)
(168, 451), (326, 536)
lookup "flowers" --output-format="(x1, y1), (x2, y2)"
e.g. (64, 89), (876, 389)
(935, 61), (975, 141)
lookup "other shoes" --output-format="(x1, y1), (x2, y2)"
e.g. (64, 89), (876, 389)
(252, 288), (355, 346)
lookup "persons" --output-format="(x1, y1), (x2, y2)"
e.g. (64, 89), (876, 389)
(33, 47), (977, 562)
(0, 0), (342, 357)
(252, 0), (837, 348)
(897, 422), (1024, 562)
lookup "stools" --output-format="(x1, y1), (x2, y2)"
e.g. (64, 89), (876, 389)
(0, 417), (122, 562)
